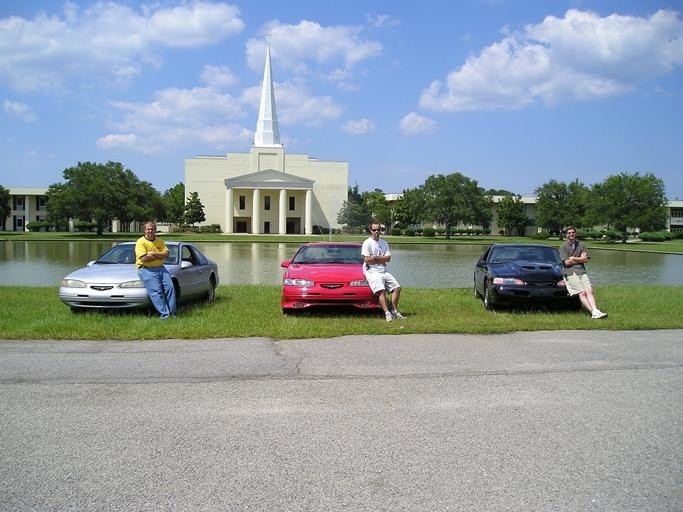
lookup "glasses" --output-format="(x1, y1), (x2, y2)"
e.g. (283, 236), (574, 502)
(370, 228), (381, 232)
(566, 232), (576, 235)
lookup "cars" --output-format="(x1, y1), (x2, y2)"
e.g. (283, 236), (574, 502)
(474, 243), (581, 310)
(282, 243), (382, 310)
(59, 241), (219, 311)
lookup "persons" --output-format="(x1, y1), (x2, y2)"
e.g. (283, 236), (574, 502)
(559, 225), (607, 319)
(134, 220), (178, 320)
(361, 222), (403, 321)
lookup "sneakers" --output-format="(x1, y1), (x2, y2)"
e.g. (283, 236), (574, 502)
(591, 310), (603, 319)
(384, 311), (394, 322)
(595, 309), (608, 317)
(391, 309), (407, 320)
